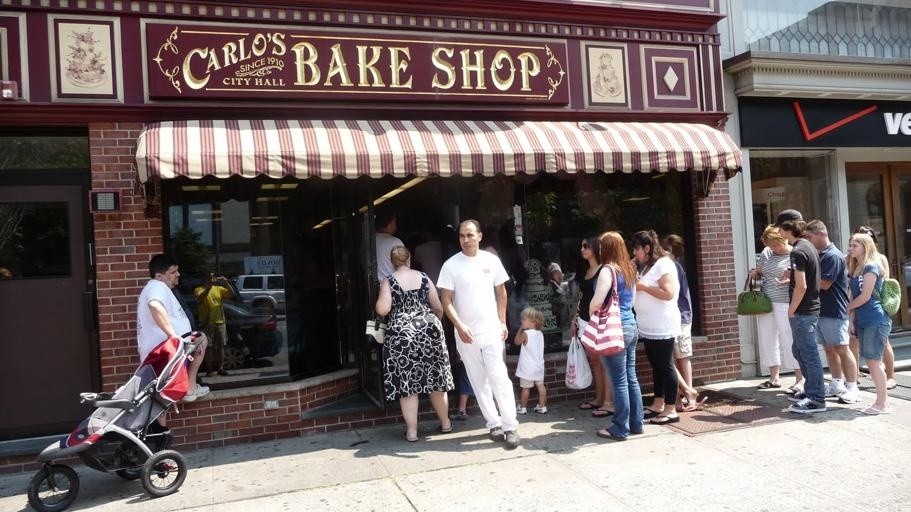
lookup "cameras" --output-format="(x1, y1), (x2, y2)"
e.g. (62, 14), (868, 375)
(216, 276), (223, 281)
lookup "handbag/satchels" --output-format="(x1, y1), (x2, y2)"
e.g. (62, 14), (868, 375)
(363, 302), (391, 352)
(737, 267), (774, 316)
(581, 293), (626, 358)
(879, 276), (902, 318)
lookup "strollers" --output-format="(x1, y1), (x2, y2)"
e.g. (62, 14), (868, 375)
(23, 329), (211, 512)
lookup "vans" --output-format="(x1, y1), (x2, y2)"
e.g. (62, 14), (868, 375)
(230, 274), (286, 316)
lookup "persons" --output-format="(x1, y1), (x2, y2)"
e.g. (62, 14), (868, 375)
(513, 305), (550, 415)
(374, 245), (453, 442)
(631, 229), (682, 425)
(413, 222), (445, 298)
(657, 234), (709, 413)
(374, 206), (411, 287)
(441, 229), (495, 421)
(748, 208), (897, 416)
(193, 271), (235, 377)
(135, 254), (210, 474)
(435, 218), (520, 450)
(572, 233), (615, 418)
(588, 231), (644, 440)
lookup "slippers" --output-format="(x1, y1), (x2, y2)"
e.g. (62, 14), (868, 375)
(683, 397), (710, 411)
(442, 422), (455, 435)
(757, 381), (781, 389)
(598, 427), (627, 442)
(579, 402), (603, 410)
(593, 407), (617, 418)
(649, 415), (679, 426)
(858, 405), (893, 416)
(643, 408), (661, 419)
(884, 379), (896, 390)
(785, 386), (804, 395)
(403, 432), (419, 445)
(183, 384), (211, 402)
(676, 399), (684, 412)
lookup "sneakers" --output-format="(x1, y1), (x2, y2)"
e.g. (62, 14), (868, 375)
(790, 398), (827, 414)
(516, 403), (528, 415)
(505, 430), (520, 449)
(533, 404), (548, 414)
(825, 383), (843, 396)
(489, 427), (504, 440)
(787, 391), (805, 401)
(839, 389), (862, 404)
(450, 411), (469, 421)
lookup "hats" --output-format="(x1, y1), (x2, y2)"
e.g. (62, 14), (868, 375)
(772, 208), (805, 227)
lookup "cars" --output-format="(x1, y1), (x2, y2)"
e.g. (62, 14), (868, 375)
(180, 294), (284, 375)
